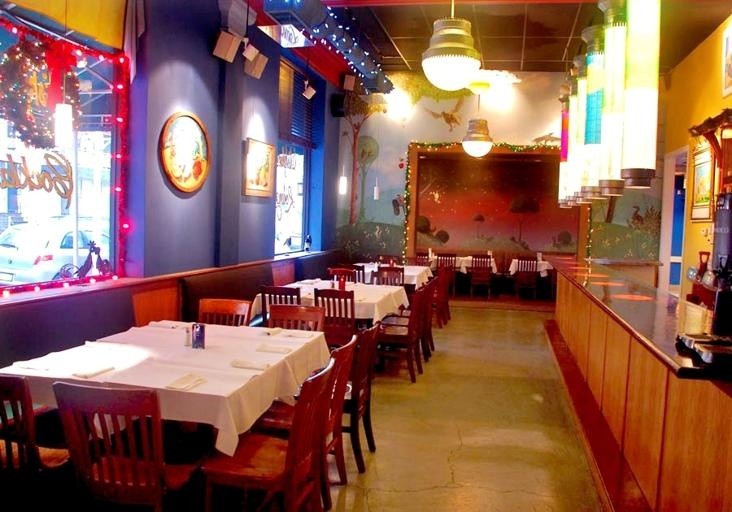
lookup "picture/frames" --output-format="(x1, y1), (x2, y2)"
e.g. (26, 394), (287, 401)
(158, 111), (212, 193)
(243, 137), (275, 198)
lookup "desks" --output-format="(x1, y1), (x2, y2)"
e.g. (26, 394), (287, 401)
(510, 259), (553, 277)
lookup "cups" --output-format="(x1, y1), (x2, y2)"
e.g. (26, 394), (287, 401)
(428, 247), (433, 257)
(488, 251), (492, 259)
(536, 252), (542, 262)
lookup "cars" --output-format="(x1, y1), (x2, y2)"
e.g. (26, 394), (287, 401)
(0, 217), (111, 288)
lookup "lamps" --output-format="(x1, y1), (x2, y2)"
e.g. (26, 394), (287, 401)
(374, 177), (380, 200)
(242, 0), (260, 62)
(301, 47), (316, 100)
(422, 0), (481, 92)
(556, 0), (661, 209)
(338, 165), (347, 195)
(462, 93), (493, 158)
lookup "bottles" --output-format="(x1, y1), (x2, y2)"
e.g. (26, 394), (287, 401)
(378, 261), (381, 267)
(374, 261), (378, 269)
(393, 262), (396, 267)
(305, 234), (311, 253)
(183, 326), (191, 347)
(331, 281), (336, 288)
